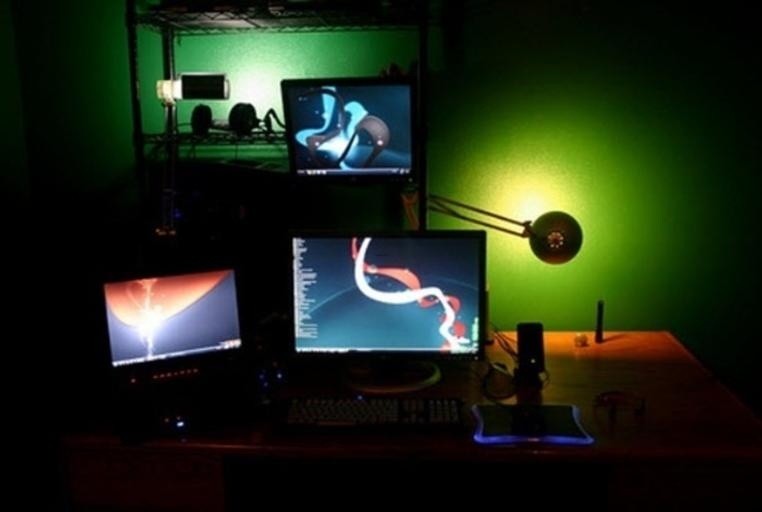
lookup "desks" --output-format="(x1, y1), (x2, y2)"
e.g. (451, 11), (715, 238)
(43, 320), (760, 469)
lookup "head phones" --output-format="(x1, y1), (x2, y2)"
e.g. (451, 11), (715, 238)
(191, 103), (260, 133)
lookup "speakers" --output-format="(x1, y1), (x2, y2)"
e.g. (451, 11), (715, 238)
(517, 323), (546, 374)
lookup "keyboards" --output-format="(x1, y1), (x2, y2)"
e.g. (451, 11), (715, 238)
(289, 397), (462, 426)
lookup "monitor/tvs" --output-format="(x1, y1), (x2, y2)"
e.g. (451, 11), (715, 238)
(281, 73), (414, 177)
(288, 230), (487, 395)
(102, 267), (242, 368)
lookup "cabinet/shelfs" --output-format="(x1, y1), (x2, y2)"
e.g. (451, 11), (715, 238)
(120, 0), (430, 317)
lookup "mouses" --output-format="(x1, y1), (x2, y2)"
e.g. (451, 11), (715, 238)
(511, 403), (546, 435)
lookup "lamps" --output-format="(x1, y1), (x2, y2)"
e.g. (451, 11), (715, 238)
(425, 188), (584, 266)
(148, 67), (235, 109)
